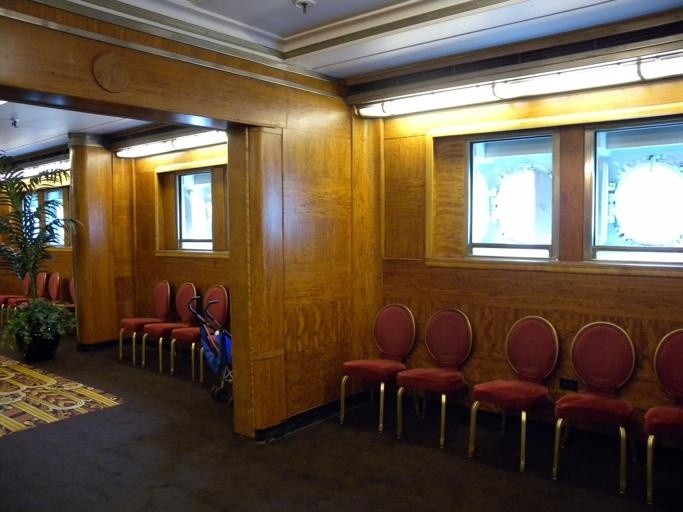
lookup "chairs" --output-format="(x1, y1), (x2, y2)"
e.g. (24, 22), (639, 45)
(644, 322), (682, 505)
(468, 316), (559, 472)
(339, 300), (413, 436)
(549, 321), (636, 497)
(2, 271), (76, 328)
(394, 309), (472, 453)
(118, 281), (233, 389)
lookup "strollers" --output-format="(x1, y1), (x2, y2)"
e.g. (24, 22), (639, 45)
(187, 295), (233, 405)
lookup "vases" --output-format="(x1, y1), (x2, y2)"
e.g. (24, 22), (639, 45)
(12, 329), (60, 361)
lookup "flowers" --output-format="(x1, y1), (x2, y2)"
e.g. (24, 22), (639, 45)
(0, 297), (81, 356)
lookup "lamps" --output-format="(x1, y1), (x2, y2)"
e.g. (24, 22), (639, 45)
(345, 36), (682, 118)
(110, 126), (228, 158)
(0, 154), (70, 182)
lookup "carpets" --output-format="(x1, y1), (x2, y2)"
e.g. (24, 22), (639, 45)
(0, 355), (124, 440)
